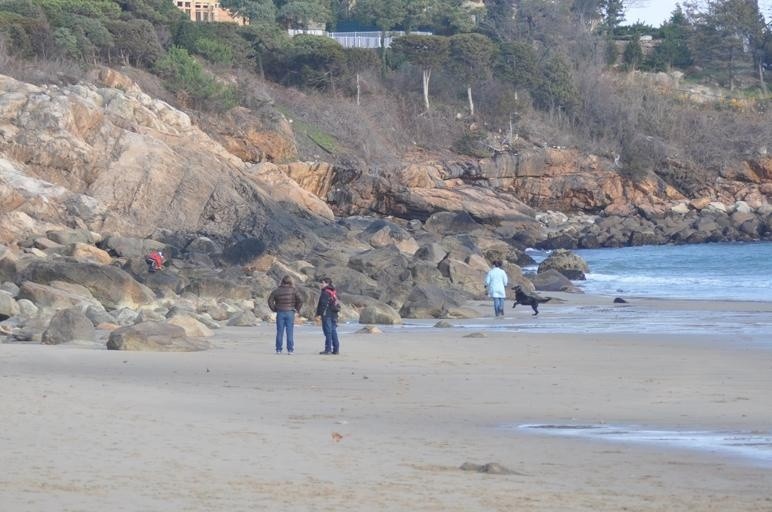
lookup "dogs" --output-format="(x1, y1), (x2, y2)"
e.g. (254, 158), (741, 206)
(511, 285), (552, 315)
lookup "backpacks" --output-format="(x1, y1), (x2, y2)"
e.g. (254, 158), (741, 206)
(324, 288), (341, 312)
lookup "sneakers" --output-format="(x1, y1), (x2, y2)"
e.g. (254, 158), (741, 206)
(319, 350), (331, 355)
(287, 350), (295, 355)
(275, 351), (281, 355)
(331, 350), (340, 355)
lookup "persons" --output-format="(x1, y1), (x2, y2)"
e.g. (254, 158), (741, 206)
(269, 276), (302, 355)
(484, 260), (511, 314)
(317, 277), (340, 352)
(144, 251), (164, 273)
